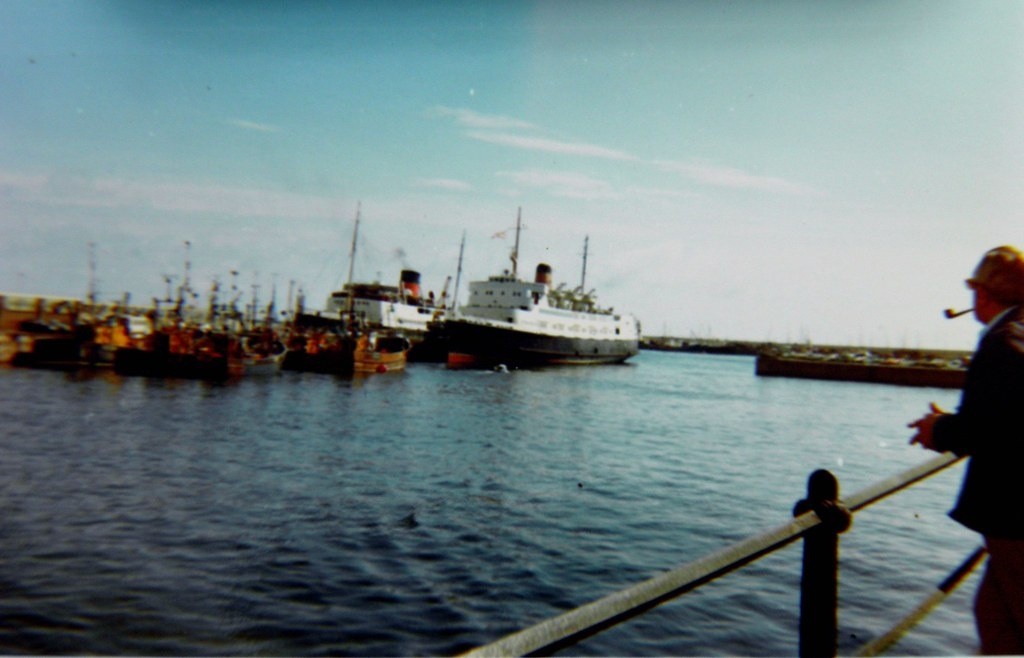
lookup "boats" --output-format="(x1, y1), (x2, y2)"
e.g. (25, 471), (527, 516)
(427, 204), (641, 371)
(315, 200), (468, 337)
(771, 322), (970, 370)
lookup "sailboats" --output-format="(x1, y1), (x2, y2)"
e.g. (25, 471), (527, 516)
(1, 243), (412, 380)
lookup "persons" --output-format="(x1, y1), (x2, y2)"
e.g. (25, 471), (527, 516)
(908, 246), (1024, 658)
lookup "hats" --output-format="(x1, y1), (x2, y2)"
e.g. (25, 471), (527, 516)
(964, 245), (1024, 300)
